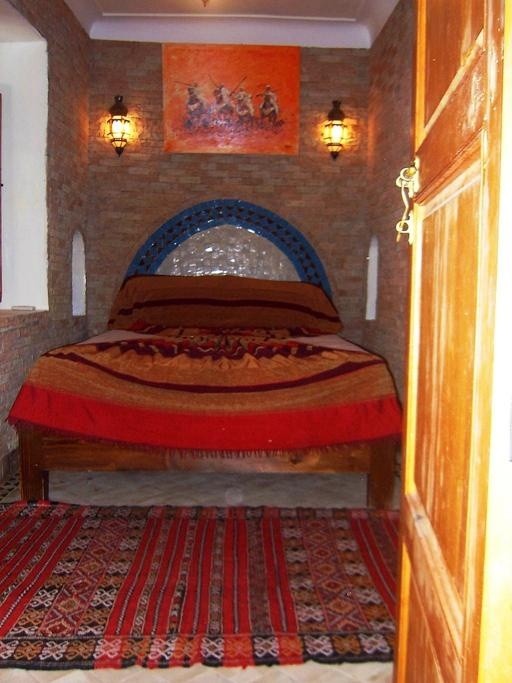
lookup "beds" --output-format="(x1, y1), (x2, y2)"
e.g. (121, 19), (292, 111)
(17, 196), (404, 511)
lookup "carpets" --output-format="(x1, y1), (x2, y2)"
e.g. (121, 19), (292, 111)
(1, 498), (401, 673)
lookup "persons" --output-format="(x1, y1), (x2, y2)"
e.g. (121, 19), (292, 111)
(183, 80), (282, 131)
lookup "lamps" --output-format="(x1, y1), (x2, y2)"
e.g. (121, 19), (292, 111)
(105, 94), (132, 158)
(318, 98), (351, 161)
